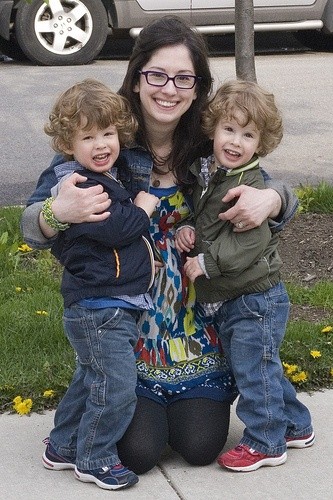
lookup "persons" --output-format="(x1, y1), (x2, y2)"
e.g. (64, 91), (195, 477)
(172, 76), (319, 474)
(40, 80), (163, 490)
(18, 14), (302, 476)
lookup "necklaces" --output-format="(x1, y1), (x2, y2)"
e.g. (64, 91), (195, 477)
(151, 173), (161, 188)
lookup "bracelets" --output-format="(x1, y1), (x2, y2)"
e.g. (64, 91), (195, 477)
(41, 197), (71, 231)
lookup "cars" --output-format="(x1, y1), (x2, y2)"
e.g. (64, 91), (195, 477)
(0, 0), (333, 66)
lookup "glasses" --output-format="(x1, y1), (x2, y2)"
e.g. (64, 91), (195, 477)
(137, 69), (202, 89)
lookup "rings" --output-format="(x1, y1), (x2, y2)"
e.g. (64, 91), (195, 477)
(236, 221), (244, 230)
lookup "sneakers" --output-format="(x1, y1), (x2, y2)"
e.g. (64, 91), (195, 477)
(41, 436), (76, 471)
(284, 432), (315, 449)
(217, 443), (288, 472)
(74, 464), (139, 490)
